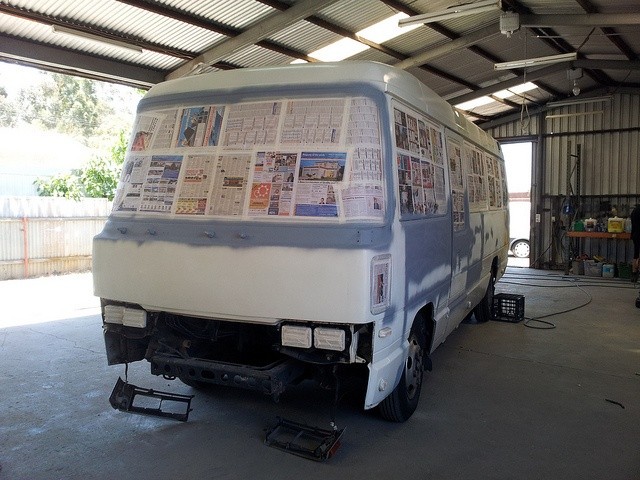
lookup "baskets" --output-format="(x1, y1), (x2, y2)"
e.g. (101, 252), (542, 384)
(493, 292), (525, 323)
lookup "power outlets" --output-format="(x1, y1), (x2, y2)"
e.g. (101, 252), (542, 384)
(536, 214), (540, 222)
(552, 216), (555, 223)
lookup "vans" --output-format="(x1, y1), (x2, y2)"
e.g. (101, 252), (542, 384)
(90, 59), (511, 463)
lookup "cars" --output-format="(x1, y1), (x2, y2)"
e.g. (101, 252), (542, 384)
(507, 225), (531, 259)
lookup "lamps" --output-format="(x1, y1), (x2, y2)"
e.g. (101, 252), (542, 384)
(572, 80), (581, 97)
(545, 111), (604, 119)
(546, 96), (614, 108)
(398, 0), (502, 28)
(494, 51), (578, 71)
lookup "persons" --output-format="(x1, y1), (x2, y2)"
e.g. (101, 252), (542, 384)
(319, 197), (325, 203)
(286, 173), (293, 181)
(273, 172), (282, 183)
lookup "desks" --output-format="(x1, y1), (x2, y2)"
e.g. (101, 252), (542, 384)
(566, 231), (633, 278)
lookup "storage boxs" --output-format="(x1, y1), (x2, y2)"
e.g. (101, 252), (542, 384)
(584, 261), (602, 277)
(602, 265), (615, 279)
(571, 261), (584, 275)
